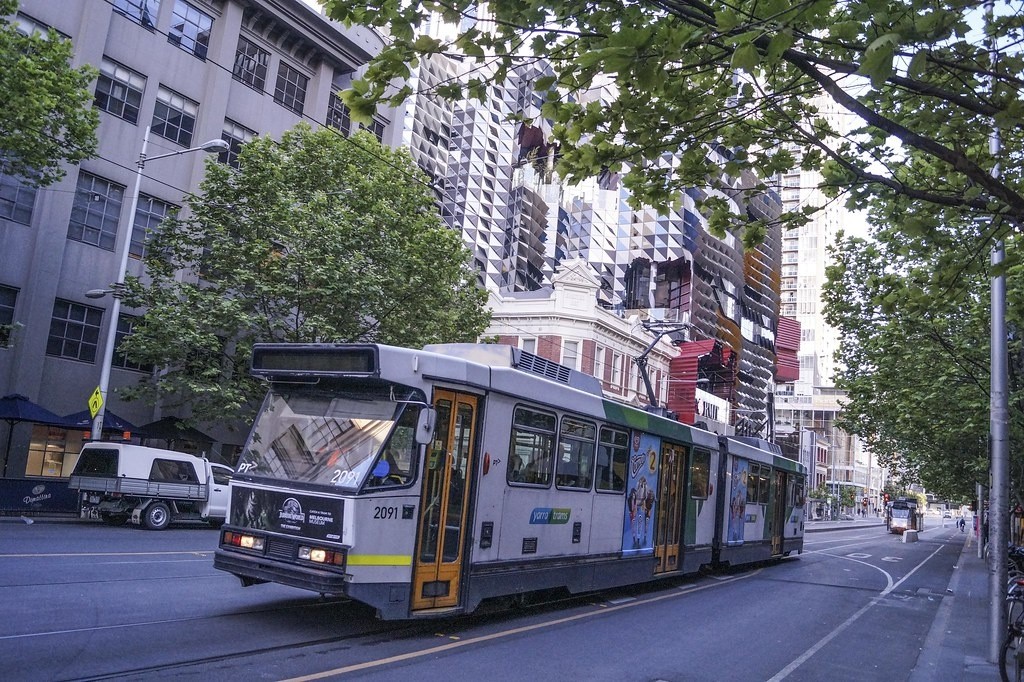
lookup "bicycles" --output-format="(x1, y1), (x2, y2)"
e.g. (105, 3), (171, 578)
(982, 537), (1024, 682)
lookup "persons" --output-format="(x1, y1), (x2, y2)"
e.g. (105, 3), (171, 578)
(959, 518), (965, 530)
(508, 444), (569, 484)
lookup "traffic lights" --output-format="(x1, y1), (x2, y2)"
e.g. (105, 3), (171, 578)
(863, 501), (867, 505)
(884, 494), (890, 502)
(851, 495), (854, 499)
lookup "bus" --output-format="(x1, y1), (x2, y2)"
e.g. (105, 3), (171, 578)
(885, 491), (923, 535)
(211, 317), (810, 628)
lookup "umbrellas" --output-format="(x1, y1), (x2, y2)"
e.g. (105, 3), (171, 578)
(1, 393), (221, 481)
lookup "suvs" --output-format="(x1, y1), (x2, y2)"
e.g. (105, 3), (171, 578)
(944, 511), (951, 519)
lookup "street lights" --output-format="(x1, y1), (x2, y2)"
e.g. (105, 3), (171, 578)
(85, 124), (229, 444)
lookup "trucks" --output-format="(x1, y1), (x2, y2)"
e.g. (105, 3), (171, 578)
(67, 438), (235, 531)
